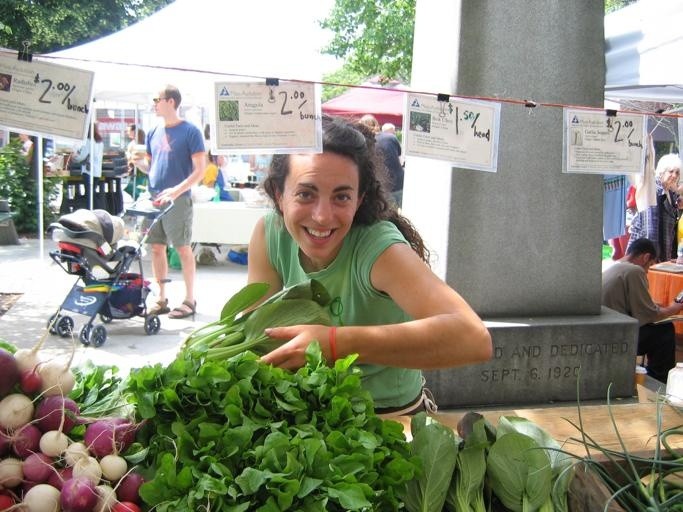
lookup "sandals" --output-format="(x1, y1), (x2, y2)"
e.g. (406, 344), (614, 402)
(167, 299), (198, 319)
(139, 299), (171, 317)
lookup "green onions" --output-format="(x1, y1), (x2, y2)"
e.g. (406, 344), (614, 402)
(520, 364), (683, 511)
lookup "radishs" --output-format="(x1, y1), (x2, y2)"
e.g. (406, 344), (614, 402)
(0, 311), (128, 511)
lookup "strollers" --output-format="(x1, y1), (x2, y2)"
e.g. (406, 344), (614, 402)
(46, 196), (175, 347)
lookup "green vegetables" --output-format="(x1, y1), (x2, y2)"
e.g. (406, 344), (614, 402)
(398, 414), (575, 511)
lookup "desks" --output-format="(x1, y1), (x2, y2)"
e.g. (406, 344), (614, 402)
(647, 259), (683, 338)
(189, 199), (273, 244)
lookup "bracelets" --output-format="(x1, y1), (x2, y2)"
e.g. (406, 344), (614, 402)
(326, 324), (338, 363)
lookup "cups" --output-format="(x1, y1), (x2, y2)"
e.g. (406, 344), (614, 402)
(133, 145), (146, 162)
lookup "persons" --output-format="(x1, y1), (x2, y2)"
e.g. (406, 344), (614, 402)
(601, 152), (683, 385)
(379, 122), (395, 136)
(358, 113), (404, 191)
(0, 121), (273, 202)
(127, 82), (208, 319)
(239, 110), (495, 413)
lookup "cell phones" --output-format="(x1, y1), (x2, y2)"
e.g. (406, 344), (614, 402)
(675, 291), (683, 303)
